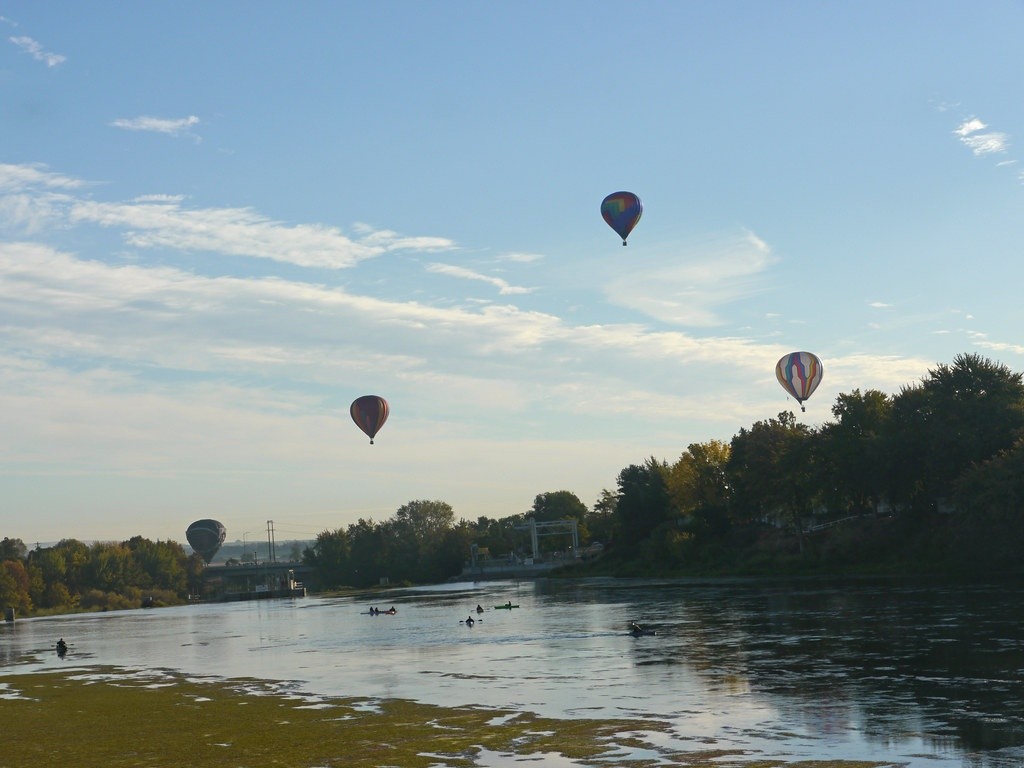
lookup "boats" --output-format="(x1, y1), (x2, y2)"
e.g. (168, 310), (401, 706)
(494, 604), (519, 610)
(55, 644), (67, 651)
(466, 620), (474, 626)
(476, 609), (483, 613)
(385, 610), (395, 615)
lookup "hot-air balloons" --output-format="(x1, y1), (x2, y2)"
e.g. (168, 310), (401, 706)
(185, 519), (228, 567)
(599, 191), (644, 247)
(774, 350), (824, 413)
(349, 393), (390, 445)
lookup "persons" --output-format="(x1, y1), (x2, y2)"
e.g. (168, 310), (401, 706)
(389, 606), (395, 611)
(370, 606), (374, 612)
(477, 605), (482, 610)
(375, 607), (378, 611)
(57, 638), (66, 647)
(465, 616), (474, 623)
(508, 601), (511, 606)
